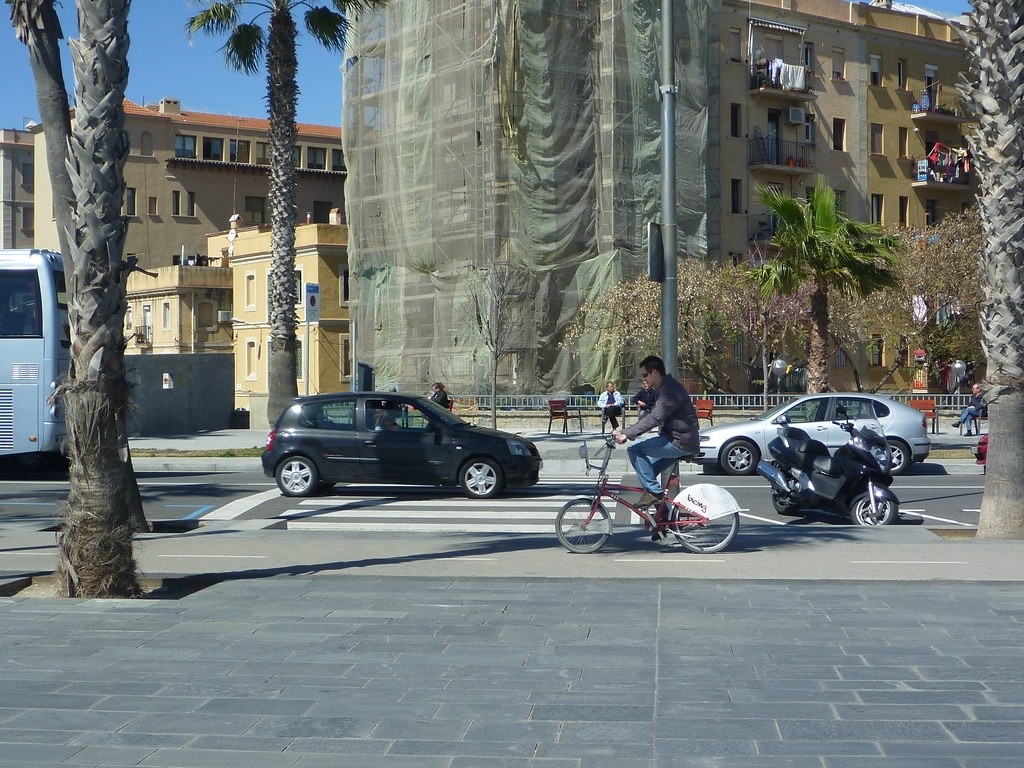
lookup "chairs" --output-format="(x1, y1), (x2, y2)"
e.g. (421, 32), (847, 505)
(446, 399), (454, 412)
(601, 408), (625, 434)
(960, 409), (988, 436)
(909, 400), (939, 435)
(694, 400), (714, 426)
(548, 400), (582, 436)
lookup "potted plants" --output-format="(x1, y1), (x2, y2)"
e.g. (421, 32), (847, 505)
(787, 156), (795, 167)
(798, 158), (807, 167)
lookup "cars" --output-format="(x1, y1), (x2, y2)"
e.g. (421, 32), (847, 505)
(261, 391), (543, 499)
(692, 393), (930, 475)
(971, 434), (988, 465)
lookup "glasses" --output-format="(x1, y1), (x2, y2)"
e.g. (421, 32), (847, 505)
(642, 372), (649, 378)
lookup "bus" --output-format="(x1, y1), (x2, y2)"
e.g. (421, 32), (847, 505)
(0, 248), (71, 456)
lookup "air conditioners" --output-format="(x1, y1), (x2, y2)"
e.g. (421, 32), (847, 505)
(218, 311), (232, 322)
(785, 107), (804, 126)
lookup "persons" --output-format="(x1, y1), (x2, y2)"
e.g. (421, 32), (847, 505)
(348, 403), (354, 424)
(951, 383), (985, 437)
(372, 401), (409, 432)
(611, 355), (700, 541)
(631, 378), (657, 419)
(597, 382), (625, 433)
(430, 382), (447, 409)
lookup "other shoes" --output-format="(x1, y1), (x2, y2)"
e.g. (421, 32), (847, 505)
(602, 417), (608, 423)
(964, 432), (972, 437)
(951, 423), (959, 428)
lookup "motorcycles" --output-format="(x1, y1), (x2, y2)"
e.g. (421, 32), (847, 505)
(757, 404), (900, 526)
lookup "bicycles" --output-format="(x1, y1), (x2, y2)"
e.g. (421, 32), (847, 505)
(555, 431), (751, 554)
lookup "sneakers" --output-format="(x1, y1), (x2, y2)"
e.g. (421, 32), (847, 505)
(633, 490), (665, 508)
(652, 516), (680, 541)
